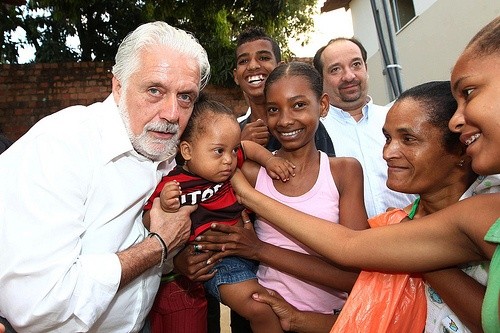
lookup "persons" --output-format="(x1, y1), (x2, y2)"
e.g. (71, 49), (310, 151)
(141, 91), (296, 333)
(0, 20), (210, 333)
(174, 59), (370, 333)
(229, 15), (500, 333)
(229, 29), (335, 158)
(312, 37), (421, 222)
(249, 81), (487, 333)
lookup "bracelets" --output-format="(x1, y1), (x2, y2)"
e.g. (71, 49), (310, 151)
(147, 232), (168, 268)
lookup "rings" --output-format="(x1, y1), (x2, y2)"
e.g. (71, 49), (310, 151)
(221, 243), (226, 252)
(244, 220), (250, 223)
